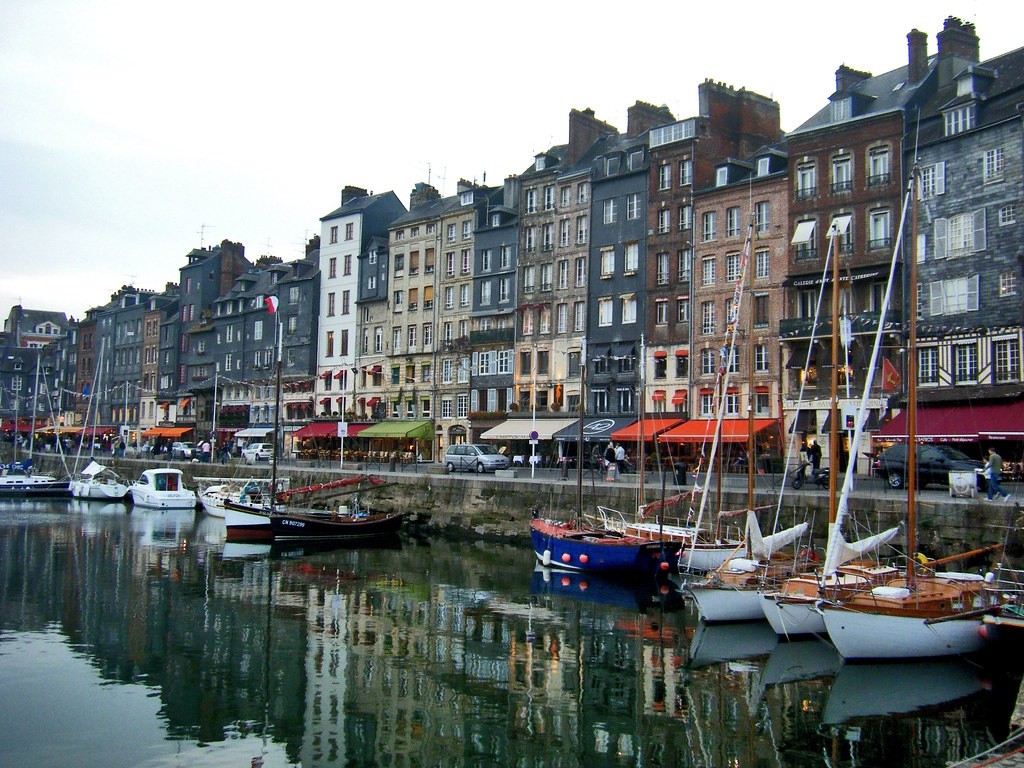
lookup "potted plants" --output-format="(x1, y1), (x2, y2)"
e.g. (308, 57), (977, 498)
(550, 402), (560, 412)
(576, 403), (585, 412)
(467, 410), (508, 428)
(509, 403), (518, 412)
(531, 403), (539, 411)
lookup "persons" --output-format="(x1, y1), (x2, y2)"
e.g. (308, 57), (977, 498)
(800, 439), (822, 478)
(592, 443), (747, 474)
(974, 448), (1011, 504)
(16, 433), (234, 465)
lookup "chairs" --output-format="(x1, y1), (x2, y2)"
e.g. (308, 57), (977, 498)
(299, 449), (414, 464)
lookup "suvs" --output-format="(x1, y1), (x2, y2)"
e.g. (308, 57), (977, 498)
(442, 444), (511, 473)
(872, 445), (995, 490)
(240, 442), (275, 461)
(171, 442), (202, 459)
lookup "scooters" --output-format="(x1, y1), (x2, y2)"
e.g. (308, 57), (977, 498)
(791, 462), (831, 489)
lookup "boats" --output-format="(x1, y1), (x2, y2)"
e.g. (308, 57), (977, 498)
(265, 507), (404, 540)
(197, 482), (288, 518)
(129, 468), (197, 509)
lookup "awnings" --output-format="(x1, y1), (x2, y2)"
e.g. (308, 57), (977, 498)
(1, 423), (115, 436)
(479, 419), (779, 442)
(320, 366), (382, 407)
(790, 220), (817, 244)
(292, 421), (435, 440)
(179, 398), (190, 407)
(784, 346), (886, 434)
(871, 398), (1024, 442)
(141, 427), (194, 437)
(652, 390), (666, 400)
(160, 402), (169, 409)
(234, 427), (275, 437)
(825, 216), (852, 239)
(672, 390), (687, 404)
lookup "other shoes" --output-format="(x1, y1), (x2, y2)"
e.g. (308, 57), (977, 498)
(984, 497), (994, 502)
(1004, 494), (1010, 502)
(996, 492), (1000, 498)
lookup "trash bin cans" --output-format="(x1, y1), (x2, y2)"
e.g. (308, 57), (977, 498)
(674, 463), (686, 485)
(167, 450), (171, 461)
(180, 450), (185, 462)
(191, 450), (196, 462)
(389, 457), (396, 472)
(562, 462), (568, 477)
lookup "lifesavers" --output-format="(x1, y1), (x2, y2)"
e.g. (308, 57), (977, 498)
(801, 549), (819, 563)
(913, 552), (928, 571)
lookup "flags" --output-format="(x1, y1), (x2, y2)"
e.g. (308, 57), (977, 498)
(882, 359), (902, 392)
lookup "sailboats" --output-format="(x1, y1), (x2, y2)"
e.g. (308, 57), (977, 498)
(0, 352), (70, 488)
(223, 323), (355, 537)
(530, 165), (1002, 660)
(71, 335), (129, 498)
